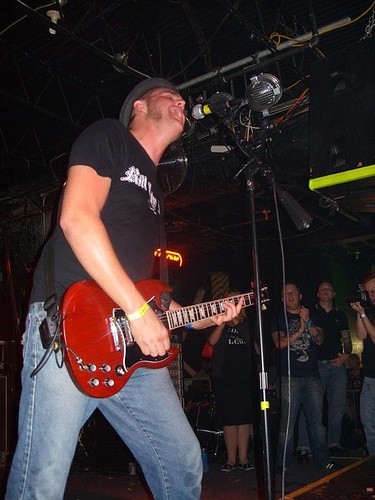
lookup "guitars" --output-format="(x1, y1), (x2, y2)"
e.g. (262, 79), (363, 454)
(60, 279), (270, 399)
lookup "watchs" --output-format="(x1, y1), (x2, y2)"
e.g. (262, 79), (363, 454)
(360, 313), (366, 318)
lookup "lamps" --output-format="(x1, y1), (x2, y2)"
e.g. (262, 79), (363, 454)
(112, 51), (129, 73)
(45, 0), (69, 35)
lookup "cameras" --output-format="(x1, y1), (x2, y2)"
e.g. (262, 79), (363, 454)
(347, 283), (371, 308)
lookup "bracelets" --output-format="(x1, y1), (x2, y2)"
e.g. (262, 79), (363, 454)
(187, 323), (194, 329)
(304, 320), (312, 327)
(125, 302), (150, 320)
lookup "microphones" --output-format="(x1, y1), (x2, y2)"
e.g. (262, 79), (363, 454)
(191, 97), (244, 120)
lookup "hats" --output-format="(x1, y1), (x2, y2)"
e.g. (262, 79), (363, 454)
(119, 78), (178, 129)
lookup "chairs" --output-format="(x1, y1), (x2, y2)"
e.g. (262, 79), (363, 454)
(193, 401), (223, 456)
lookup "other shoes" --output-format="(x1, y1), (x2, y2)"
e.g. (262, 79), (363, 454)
(220, 464), (236, 472)
(322, 463), (342, 470)
(297, 449), (308, 463)
(329, 447), (349, 456)
(237, 463), (254, 470)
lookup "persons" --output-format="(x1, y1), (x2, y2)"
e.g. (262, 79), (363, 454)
(349, 272), (375, 468)
(346, 353), (364, 406)
(296, 281), (352, 464)
(4, 79), (244, 500)
(202, 289), (259, 471)
(270, 283), (339, 473)
